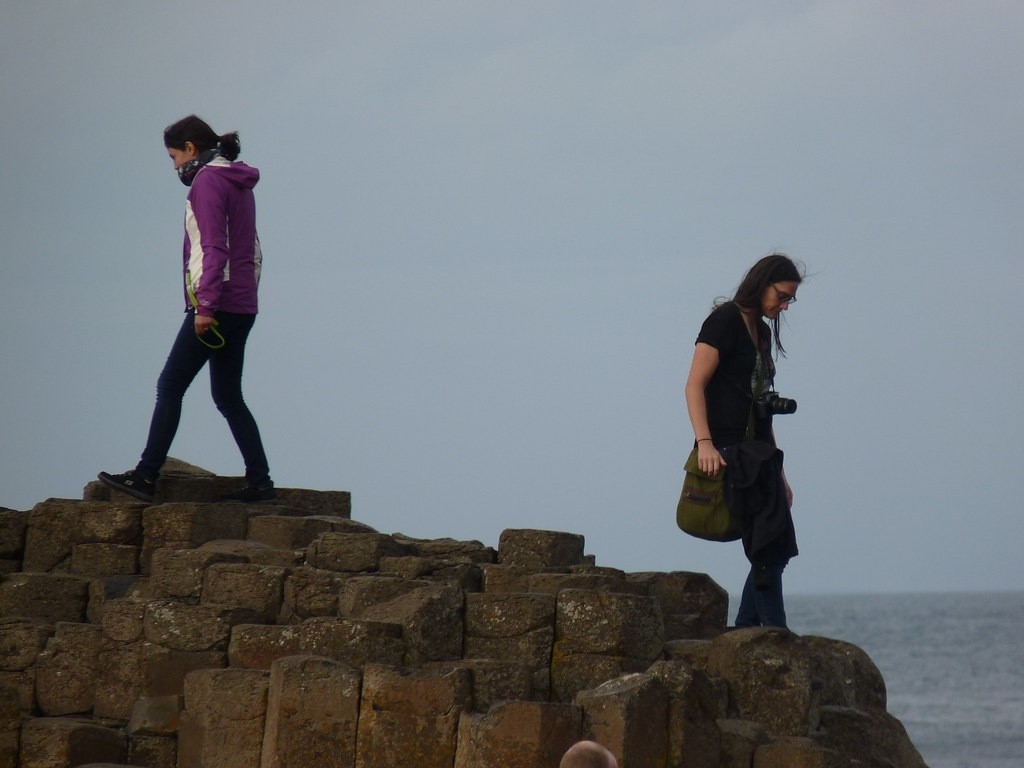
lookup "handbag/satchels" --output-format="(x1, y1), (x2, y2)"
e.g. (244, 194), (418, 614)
(676, 448), (743, 542)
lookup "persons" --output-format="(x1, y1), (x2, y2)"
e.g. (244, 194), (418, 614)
(676, 253), (805, 632)
(97, 113), (278, 504)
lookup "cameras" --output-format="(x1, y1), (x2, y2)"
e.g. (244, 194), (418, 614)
(756, 392), (797, 419)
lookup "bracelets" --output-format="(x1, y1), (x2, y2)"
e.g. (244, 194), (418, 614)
(697, 438), (712, 443)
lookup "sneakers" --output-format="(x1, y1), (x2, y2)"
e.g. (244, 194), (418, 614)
(215, 483), (277, 504)
(98, 471), (156, 502)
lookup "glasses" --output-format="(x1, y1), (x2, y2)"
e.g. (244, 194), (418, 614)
(772, 284), (797, 304)
